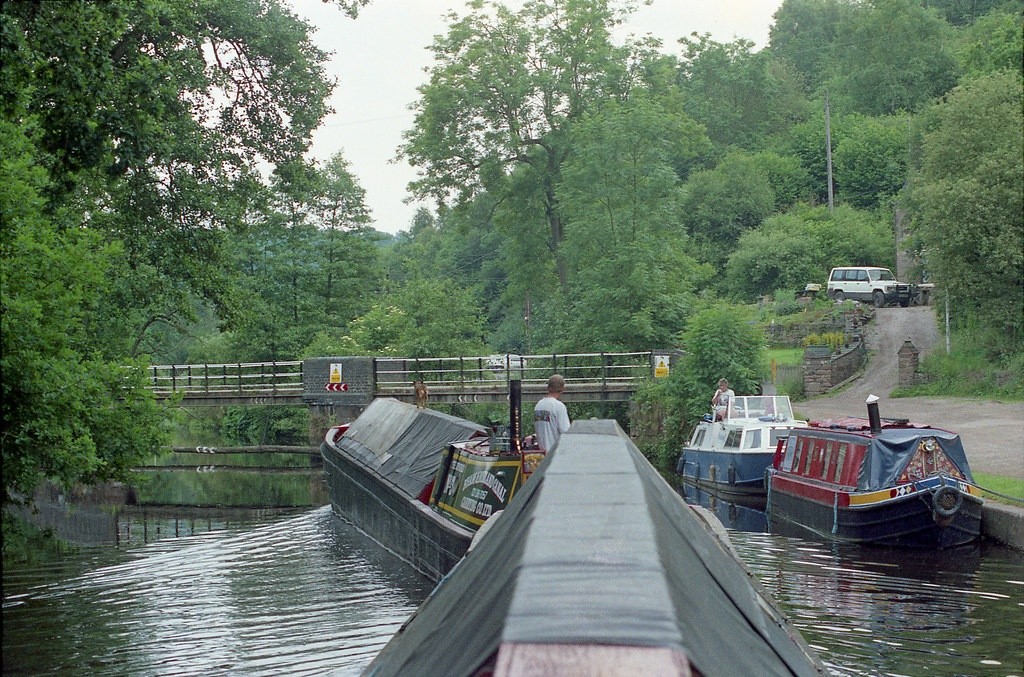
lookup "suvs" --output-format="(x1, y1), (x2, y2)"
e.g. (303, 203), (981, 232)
(827, 267), (917, 308)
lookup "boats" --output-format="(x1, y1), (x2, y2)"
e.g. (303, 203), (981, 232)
(764, 393), (984, 552)
(323, 379), (549, 588)
(677, 395), (807, 496)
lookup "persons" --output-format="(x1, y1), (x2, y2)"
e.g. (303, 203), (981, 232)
(710, 378), (735, 421)
(533, 372), (571, 455)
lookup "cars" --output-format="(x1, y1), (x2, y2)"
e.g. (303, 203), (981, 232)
(487, 353), (527, 374)
